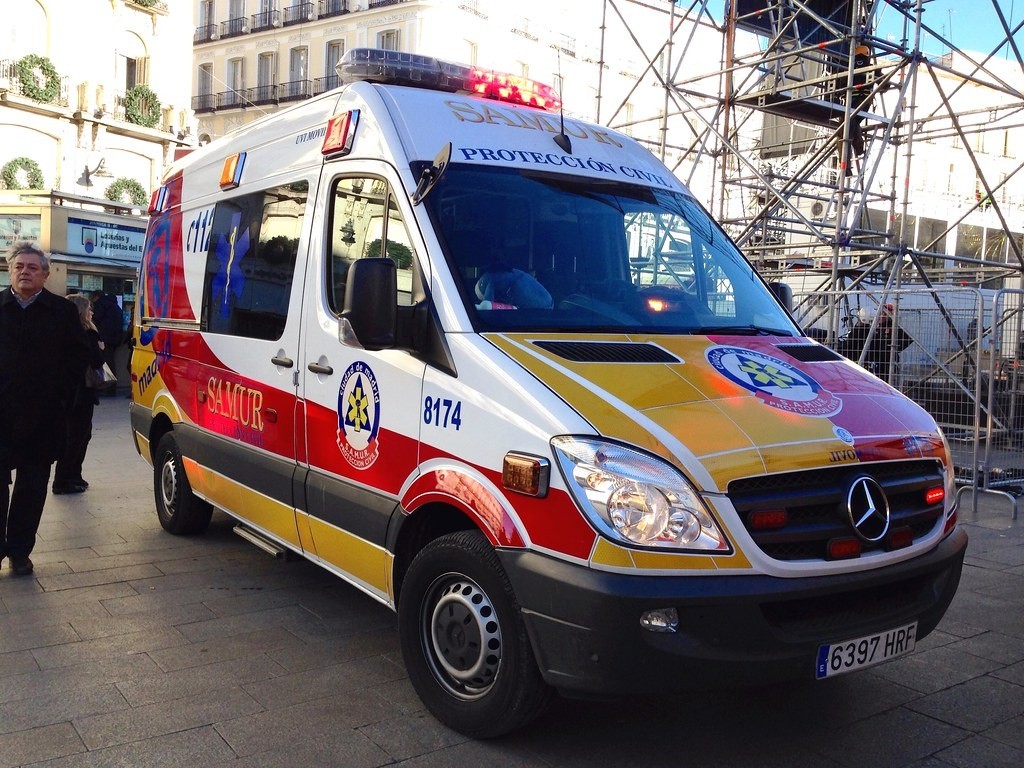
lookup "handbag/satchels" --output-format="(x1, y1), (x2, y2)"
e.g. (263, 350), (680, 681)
(73, 328), (118, 397)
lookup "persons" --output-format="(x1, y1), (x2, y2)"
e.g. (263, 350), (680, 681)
(966, 316), (986, 350)
(837, 303), (914, 389)
(0, 241), (92, 576)
(837, 45), (891, 177)
(126, 307), (134, 398)
(51, 292), (106, 494)
(88, 289), (123, 398)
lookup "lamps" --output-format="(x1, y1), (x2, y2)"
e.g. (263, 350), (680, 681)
(84, 157), (114, 187)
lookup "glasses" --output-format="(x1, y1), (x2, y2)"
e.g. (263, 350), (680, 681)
(89, 296), (96, 302)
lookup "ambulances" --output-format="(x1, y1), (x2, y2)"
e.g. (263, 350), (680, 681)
(129, 49), (971, 742)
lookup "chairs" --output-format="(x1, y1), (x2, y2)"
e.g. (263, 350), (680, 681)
(440, 216), (596, 311)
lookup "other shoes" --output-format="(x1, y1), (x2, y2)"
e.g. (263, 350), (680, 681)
(126, 393), (131, 398)
(8, 559), (33, 575)
(51, 477), (89, 493)
